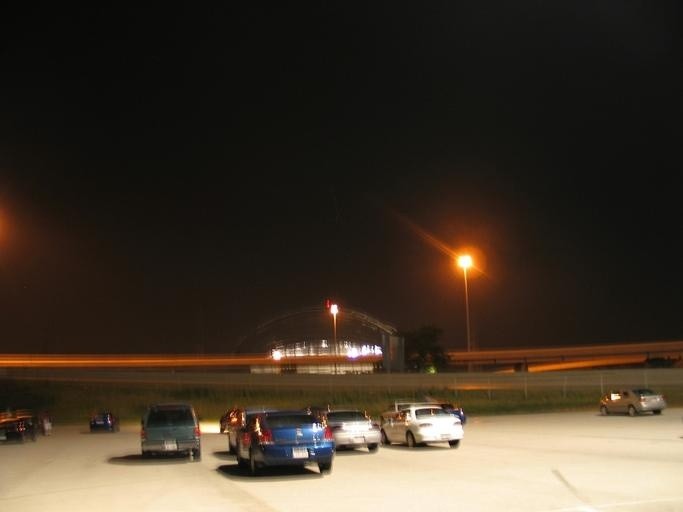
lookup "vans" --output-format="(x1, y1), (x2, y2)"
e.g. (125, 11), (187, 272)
(140, 402), (202, 463)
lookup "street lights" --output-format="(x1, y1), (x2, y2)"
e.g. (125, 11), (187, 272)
(329, 304), (340, 374)
(458, 254), (474, 371)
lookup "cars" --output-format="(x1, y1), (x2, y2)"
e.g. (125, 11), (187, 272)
(220, 399), (468, 475)
(88, 409), (121, 434)
(1, 407), (55, 444)
(598, 387), (668, 419)
